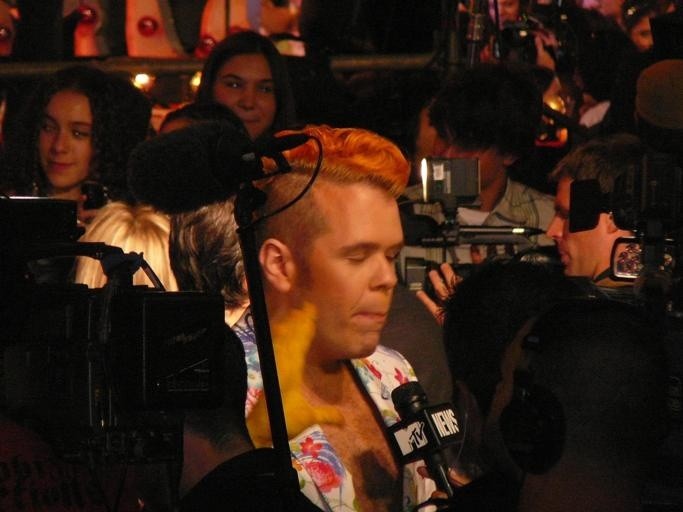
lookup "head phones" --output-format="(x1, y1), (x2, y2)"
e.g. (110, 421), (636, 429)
(498, 298), (616, 475)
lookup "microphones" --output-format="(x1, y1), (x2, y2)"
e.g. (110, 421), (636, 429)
(126, 121), (310, 215)
(384, 380), (463, 499)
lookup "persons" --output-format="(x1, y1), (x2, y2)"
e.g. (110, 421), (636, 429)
(157, 100), (249, 145)
(402, 1), (683, 320)
(194, 32), (293, 140)
(177, 324), (323, 509)
(233, 125), (445, 511)
(416, 259), (682, 510)
(488, 298), (682, 510)
(74, 202), (179, 292)
(169, 204), (251, 327)
(2, 66), (133, 225)
